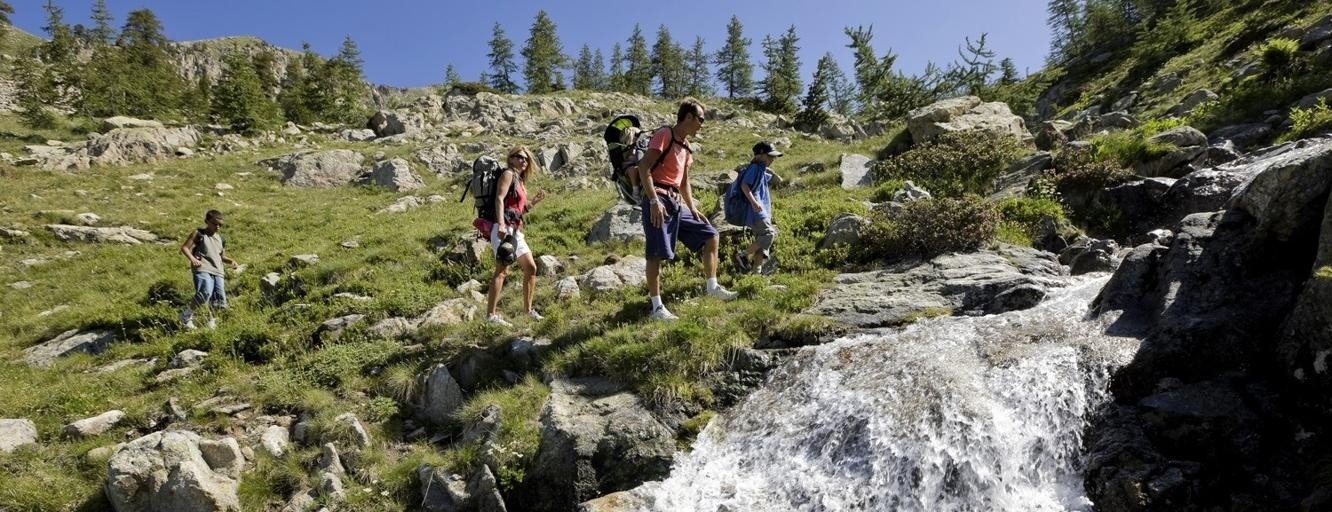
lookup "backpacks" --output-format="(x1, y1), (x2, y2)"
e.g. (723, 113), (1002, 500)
(460, 155), (524, 243)
(604, 114), (692, 211)
(725, 161), (762, 226)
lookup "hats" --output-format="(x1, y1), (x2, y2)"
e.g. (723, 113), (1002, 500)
(753, 141), (783, 157)
(496, 235), (518, 266)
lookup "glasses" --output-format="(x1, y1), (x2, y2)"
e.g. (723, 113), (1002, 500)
(513, 154), (528, 160)
(696, 114), (705, 123)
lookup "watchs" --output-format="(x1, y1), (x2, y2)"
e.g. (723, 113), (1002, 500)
(650, 199), (659, 205)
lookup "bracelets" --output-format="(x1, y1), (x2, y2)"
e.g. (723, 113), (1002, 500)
(523, 203), (533, 212)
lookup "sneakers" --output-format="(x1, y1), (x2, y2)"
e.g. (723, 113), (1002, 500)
(652, 305), (679, 321)
(736, 255), (749, 271)
(524, 309), (544, 322)
(485, 314), (513, 328)
(708, 286), (740, 302)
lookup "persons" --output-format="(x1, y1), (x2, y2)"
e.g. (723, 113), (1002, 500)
(484, 145), (545, 327)
(178, 208), (239, 326)
(638, 96), (740, 320)
(734, 141), (783, 273)
